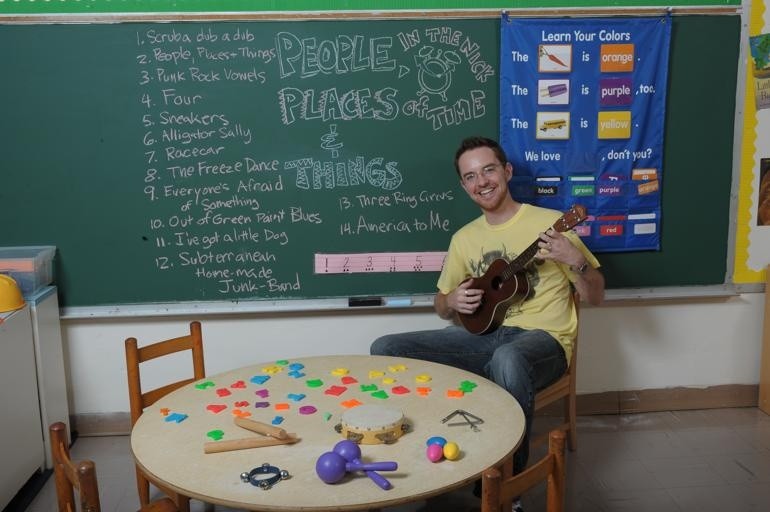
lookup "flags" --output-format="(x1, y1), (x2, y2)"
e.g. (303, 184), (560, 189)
(499, 10), (675, 257)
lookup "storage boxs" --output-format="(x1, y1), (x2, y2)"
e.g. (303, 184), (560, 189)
(0, 245), (56, 296)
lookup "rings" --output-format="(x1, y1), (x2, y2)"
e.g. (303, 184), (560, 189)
(464, 286), (470, 295)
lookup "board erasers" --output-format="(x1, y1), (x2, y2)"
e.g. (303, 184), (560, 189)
(349, 296), (382, 306)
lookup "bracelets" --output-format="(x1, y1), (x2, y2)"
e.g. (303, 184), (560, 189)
(573, 258), (591, 276)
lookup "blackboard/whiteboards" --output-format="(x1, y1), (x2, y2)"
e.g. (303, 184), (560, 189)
(0, 7), (749, 326)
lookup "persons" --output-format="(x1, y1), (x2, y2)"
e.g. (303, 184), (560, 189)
(368, 135), (606, 510)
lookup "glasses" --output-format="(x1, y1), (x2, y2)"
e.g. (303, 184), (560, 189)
(462, 161), (505, 183)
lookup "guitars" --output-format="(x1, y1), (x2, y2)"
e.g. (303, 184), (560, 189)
(456, 203), (588, 336)
